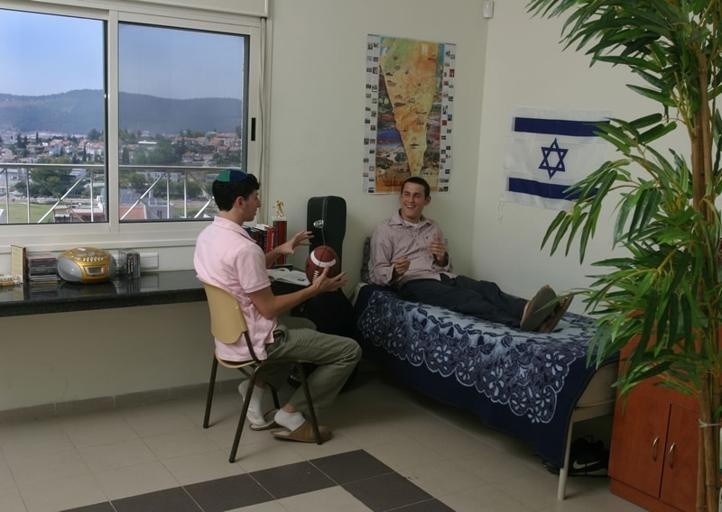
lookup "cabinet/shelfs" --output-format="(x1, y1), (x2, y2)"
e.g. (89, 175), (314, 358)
(604, 304), (721, 506)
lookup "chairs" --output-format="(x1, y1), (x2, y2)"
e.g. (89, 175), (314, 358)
(197, 273), (327, 465)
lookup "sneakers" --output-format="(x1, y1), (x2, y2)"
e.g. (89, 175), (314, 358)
(544, 437), (608, 479)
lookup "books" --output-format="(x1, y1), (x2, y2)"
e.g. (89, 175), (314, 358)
(12, 245), (57, 285)
(267, 267), (312, 287)
(118, 250), (140, 279)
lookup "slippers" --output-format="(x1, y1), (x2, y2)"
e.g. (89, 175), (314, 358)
(519, 286), (557, 331)
(249, 408), (280, 430)
(540, 294), (573, 334)
(271, 420), (332, 442)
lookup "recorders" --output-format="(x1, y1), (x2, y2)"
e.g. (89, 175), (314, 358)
(56, 246), (117, 283)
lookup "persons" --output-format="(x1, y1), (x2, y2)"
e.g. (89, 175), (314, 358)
(193, 170), (363, 443)
(367, 176), (574, 333)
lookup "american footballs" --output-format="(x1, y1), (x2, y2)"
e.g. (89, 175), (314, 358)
(305, 246), (339, 283)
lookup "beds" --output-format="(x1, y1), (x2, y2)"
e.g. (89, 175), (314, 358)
(356, 235), (616, 502)
